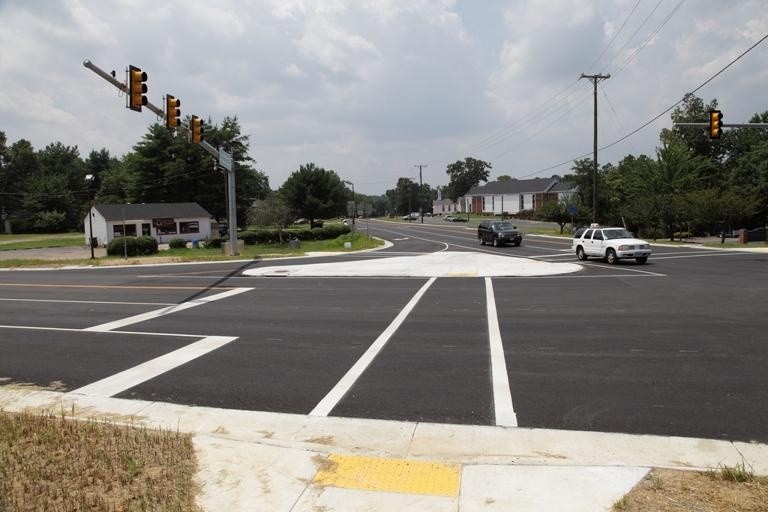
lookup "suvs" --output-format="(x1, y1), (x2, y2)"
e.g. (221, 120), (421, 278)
(478, 220), (523, 248)
(571, 223), (651, 265)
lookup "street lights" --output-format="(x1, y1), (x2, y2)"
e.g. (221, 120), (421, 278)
(85, 174), (97, 258)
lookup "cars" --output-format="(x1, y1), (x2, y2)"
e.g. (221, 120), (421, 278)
(292, 215), (350, 228)
(400, 211), (456, 222)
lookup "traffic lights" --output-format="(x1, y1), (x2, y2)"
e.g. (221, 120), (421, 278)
(165, 94), (182, 131)
(191, 115), (205, 146)
(128, 65), (148, 113)
(709, 110), (723, 139)
